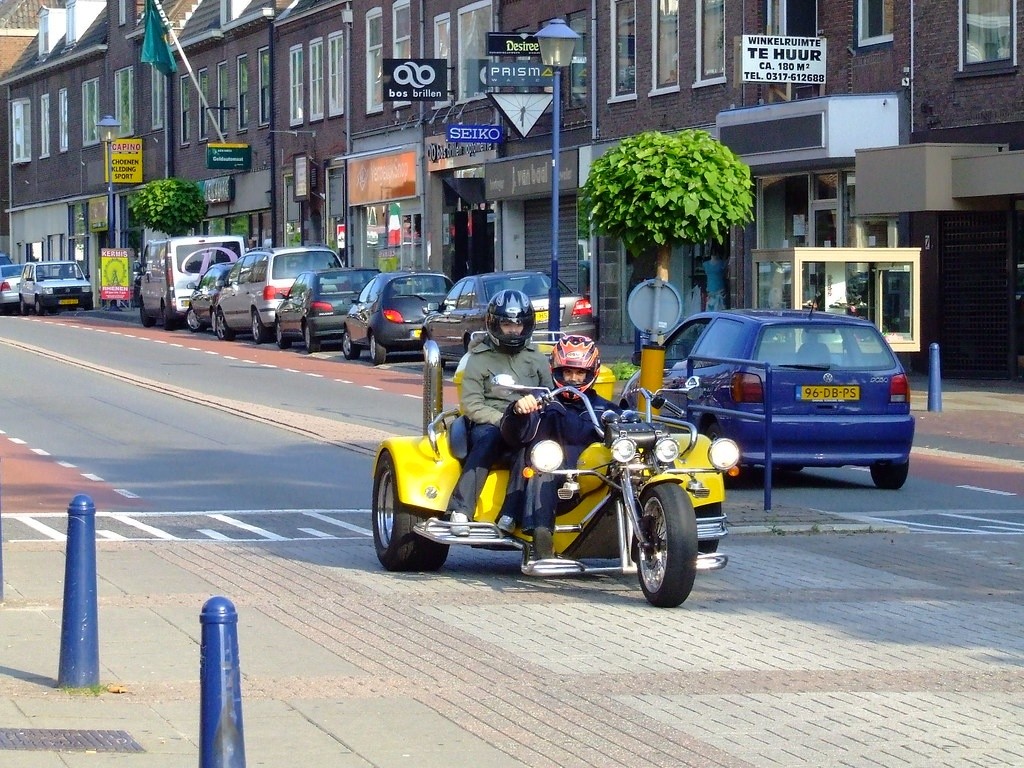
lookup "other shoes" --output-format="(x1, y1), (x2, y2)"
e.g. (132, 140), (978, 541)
(535, 527), (552, 559)
(450, 511), (470, 536)
(498, 515), (516, 534)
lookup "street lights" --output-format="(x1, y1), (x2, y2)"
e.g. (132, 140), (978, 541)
(531, 17), (582, 340)
(93, 116), (122, 311)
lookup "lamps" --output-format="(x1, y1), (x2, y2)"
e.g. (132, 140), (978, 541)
(428, 105), (464, 125)
(442, 104), (478, 126)
(415, 109), (449, 128)
(456, 99), (495, 123)
(400, 111), (436, 134)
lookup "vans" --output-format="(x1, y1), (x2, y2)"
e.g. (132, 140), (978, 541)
(137, 234), (246, 330)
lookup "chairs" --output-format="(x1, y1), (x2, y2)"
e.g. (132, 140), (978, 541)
(522, 283), (540, 297)
(797, 342), (831, 363)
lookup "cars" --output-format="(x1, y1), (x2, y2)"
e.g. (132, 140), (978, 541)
(620, 308), (915, 487)
(419, 268), (597, 367)
(0, 254), (25, 313)
(341, 271), (454, 365)
(185, 262), (235, 332)
(274, 267), (382, 353)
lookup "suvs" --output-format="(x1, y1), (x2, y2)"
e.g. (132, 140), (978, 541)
(213, 243), (343, 344)
(18, 260), (93, 315)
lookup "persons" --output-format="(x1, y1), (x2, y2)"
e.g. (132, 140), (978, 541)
(498, 336), (640, 559)
(58, 265), (69, 276)
(446, 289), (555, 536)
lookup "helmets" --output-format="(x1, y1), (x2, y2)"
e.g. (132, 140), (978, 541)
(549, 335), (600, 398)
(486, 288), (536, 351)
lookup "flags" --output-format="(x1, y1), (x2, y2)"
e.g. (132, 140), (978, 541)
(141, 0), (179, 77)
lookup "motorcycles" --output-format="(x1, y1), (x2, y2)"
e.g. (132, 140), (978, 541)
(370, 372), (743, 607)
